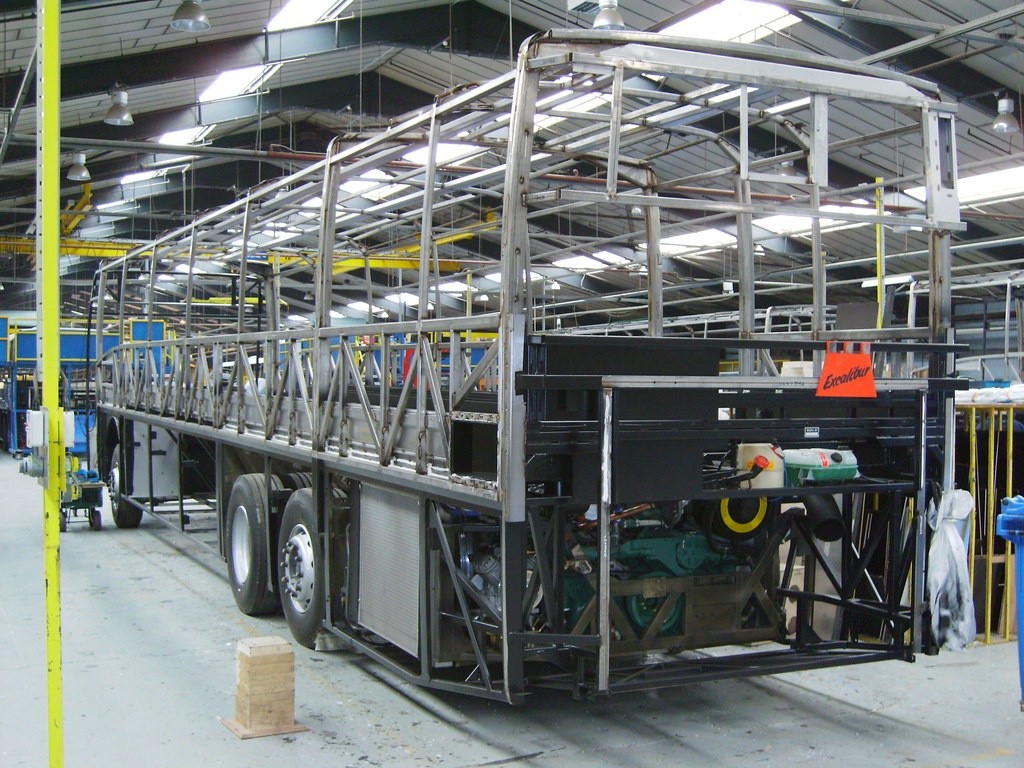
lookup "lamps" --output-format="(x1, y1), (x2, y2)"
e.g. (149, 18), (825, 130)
(170, 0), (211, 32)
(303, 294), (313, 300)
(991, 93), (1020, 134)
(376, 311), (389, 318)
(103, 88), (134, 126)
(592, 0), (626, 30)
(777, 160), (800, 177)
(630, 205), (644, 217)
(66, 152), (91, 181)
(544, 281), (560, 291)
(475, 295), (489, 302)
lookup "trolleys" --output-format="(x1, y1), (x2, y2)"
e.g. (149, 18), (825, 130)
(60, 452), (105, 532)
